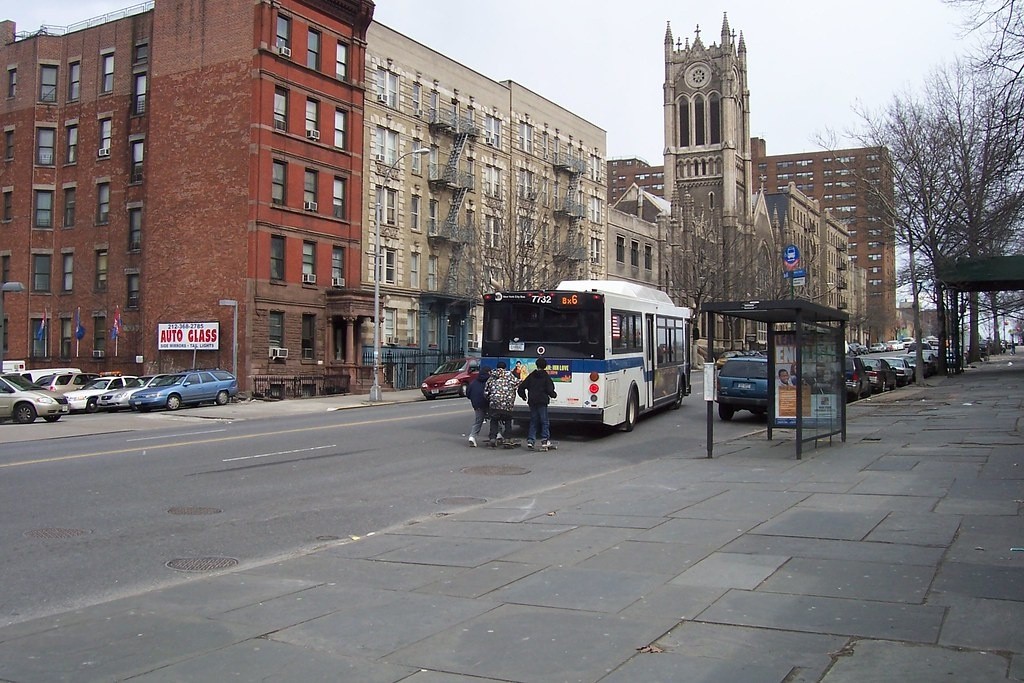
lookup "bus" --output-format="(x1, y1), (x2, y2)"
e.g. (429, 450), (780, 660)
(481, 280), (700, 432)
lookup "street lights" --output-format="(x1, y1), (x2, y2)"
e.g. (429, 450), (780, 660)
(369, 147), (429, 403)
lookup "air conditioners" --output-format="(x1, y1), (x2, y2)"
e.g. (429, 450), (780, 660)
(303, 347), (314, 360)
(307, 129), (320, 140)
(331, 276), (345, 287)
(273, 348), (289, 359)
(99, 147), (110, 157)
(93, 350), (105, 357)
(279, 45), (291, 57)
(301, 273), (316, 283)
(305, 201), (318, 211)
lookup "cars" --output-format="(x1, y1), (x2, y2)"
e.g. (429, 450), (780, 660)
(127, 370), (239, 410)
(717, 336), (938, 370)
(421, 357), (481, 400)
(0, 372), (71, 423)
(717, 339), (1012, 421)
(64, 372), (171, 413)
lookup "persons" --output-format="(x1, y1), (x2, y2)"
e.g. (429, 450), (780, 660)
(778, 369), (793, 387)
(466, 367), (504, 448)
(518, 358), (557, 447)
(484, 362), (522, 448)
(513, 361), (522, 380)
(790, 363), (797, 386)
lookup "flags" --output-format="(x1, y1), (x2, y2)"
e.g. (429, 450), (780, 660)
(111, 307), (122, 340)
(37, 309), (45, 341)
(75, 309), (85, 340)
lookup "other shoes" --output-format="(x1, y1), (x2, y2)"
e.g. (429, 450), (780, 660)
(505, 439), (514, 443)
(488, 439), (497, 448)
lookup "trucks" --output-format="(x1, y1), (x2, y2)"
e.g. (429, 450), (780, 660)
(5, 368), (82, 384)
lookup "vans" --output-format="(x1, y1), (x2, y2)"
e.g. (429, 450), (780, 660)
(34, 373), (100, 393)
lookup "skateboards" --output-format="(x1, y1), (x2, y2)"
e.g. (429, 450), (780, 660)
(502, 439), (522, 449)
(536, 442), (559, 452)
(483, 440), (505, 446)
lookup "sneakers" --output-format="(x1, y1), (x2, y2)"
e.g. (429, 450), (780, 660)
(541, 440), (552, 448)
(527, 440), (536, 449)
(469, 436), (478, 448)
(496, 433), (504, 440)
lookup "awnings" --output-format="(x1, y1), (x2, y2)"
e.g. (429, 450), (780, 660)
(937, 253), (1024, 376)
